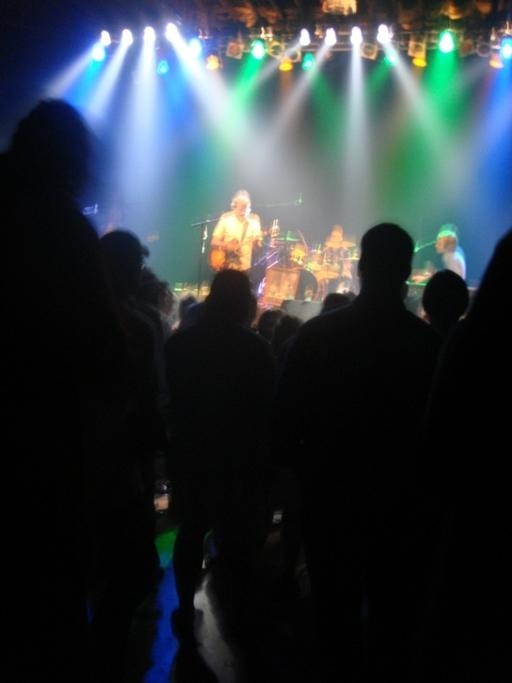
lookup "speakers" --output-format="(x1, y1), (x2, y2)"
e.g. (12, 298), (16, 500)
(282, 299), (324, 322)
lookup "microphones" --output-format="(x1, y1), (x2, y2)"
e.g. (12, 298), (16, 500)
(297, 193), (303, 205)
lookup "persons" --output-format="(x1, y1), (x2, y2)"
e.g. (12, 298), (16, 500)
(1, 102), (510, 681)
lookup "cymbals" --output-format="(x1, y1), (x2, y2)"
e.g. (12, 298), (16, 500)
(326, 240), (355, 249)
(313, 269), (339, 281)
(274, 235), (299, 241)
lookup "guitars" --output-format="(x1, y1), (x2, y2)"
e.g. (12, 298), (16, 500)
(210, 219), (279, 272)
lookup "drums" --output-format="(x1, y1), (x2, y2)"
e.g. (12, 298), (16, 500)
(290, 248), (305, 266)
(340, 258), (358, 279)
(329, 278), (356, 296)
(310, 249), (323, 263)
